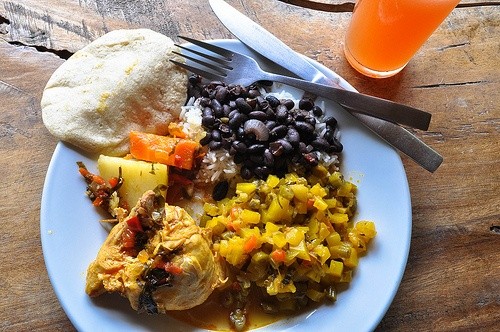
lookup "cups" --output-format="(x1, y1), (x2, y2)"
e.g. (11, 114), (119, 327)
(343, 0), (462, 79)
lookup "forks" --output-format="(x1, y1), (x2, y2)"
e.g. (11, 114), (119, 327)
(169, 34), (432, 133)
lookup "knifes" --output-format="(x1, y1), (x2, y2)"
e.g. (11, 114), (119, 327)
(209, 0), (444, 173)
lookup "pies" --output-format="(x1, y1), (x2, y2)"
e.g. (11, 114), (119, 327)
(40, 28), (188, 157)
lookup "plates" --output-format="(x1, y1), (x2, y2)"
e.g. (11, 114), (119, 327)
(40, 40), (414, 331)
